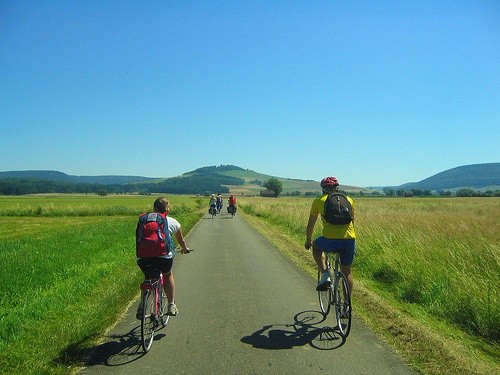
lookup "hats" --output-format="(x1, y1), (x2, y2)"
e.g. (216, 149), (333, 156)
(212, 194), (214, 196)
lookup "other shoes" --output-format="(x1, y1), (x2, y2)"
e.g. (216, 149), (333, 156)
(316, 273), (331, 291)
(168, 304), (178, 315)
(136, 309), (146, 321)
(341, 309), (349, 319)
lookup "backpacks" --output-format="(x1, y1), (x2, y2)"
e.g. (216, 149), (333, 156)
(136, 212), (171, 257)
(324, 191), (354, 225)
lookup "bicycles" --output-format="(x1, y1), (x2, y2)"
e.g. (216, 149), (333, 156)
(215, 203), (222, 213)
(209, 204), (217, 219)
(227, 202), (236, 219)
(305, 241), (353, 338)
(138, 248), (195, 353)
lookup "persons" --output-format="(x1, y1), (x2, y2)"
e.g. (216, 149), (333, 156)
(228, 196), (236, 213)
(135, 197), (190, 320)
(209, 193), (223, 216)
(304, 177), (356, 319)
